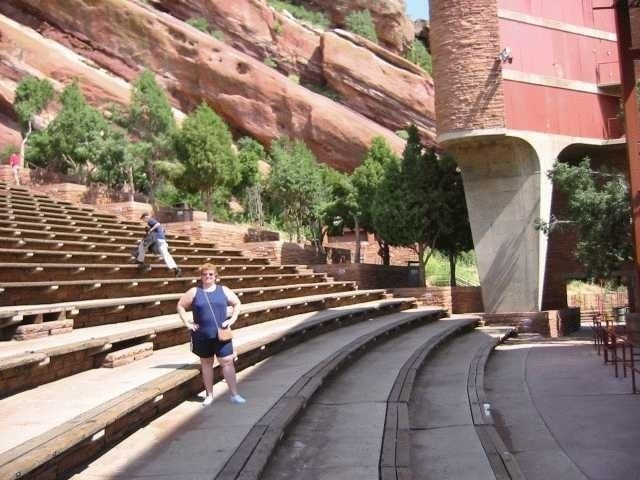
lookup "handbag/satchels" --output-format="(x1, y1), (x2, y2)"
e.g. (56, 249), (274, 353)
(218, 328), (233, 343)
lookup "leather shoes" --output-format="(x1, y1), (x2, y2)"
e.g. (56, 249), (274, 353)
(129, 260), (144, 264)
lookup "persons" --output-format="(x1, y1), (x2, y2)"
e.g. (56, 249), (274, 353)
(10, 152), (21, 186)
(176, 263), (246, 406)
(136, 212), (182, 279)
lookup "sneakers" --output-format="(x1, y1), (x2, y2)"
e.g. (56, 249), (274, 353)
(175, 269), (181, 277)
(229, 394), (245, 404)
(202, 394), (215, 406)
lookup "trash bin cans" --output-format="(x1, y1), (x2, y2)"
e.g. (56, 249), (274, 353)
(617, 306), (625, 322)
(172, 202), (193, 221)
(407, 261), (420, 287)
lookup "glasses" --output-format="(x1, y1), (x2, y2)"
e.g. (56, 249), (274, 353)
(202, 273), (213, 276)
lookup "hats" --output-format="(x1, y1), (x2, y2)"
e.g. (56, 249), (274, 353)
(140, 212), (149, 219)
